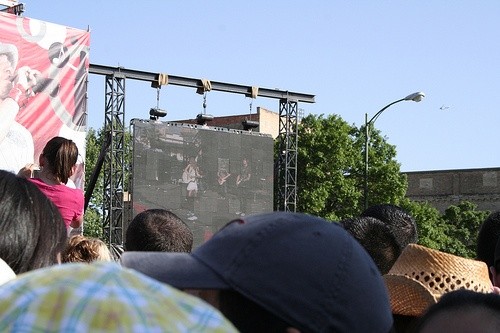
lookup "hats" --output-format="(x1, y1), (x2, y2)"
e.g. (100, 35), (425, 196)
(380, 242), (500, 316)
(0, 262), (239, 333)
(117, 212), (392, 333)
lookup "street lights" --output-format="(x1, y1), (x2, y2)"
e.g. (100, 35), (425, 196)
(363, 90), (426, 210)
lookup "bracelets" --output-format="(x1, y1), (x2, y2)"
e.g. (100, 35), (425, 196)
(8, 83), (30, 108)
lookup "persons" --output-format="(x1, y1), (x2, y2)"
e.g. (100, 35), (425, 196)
(0, 42), (41, 170)
(216, 160), (231, 200)
(184, 153), (204, 221)
(0, 136), (500, 333)
(235, 155), (252, 216)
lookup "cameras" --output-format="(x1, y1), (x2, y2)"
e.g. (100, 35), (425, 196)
(30, 167), (41, 178)
